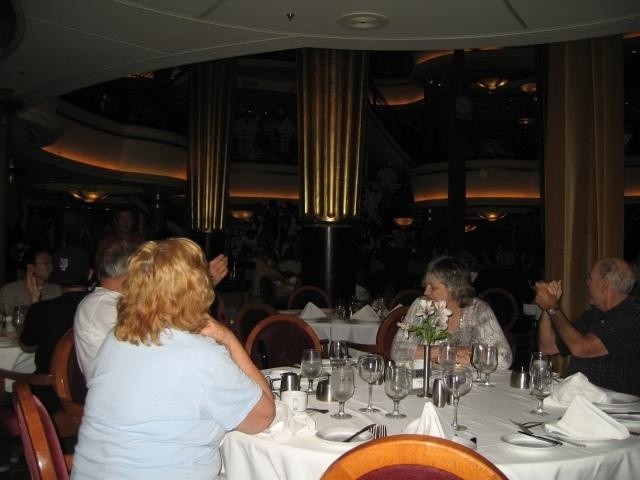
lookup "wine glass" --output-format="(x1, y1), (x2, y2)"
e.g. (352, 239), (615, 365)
(480, 346), (499, 387)
(438, 341), (456, 369)
(330, 368), (354, 420)
(301, 349), (323, 395)
(471, 345), (488, 382)
(443, 368), (473, 430)
(346, 296), (355, 322)
(335, 298), (345, 320)
(385, 366), (408, 418)
(358, 354), (385, 414)
(530, 352), (552, 380)
(531, 367), (555, 416)
(329, 339), (349, 365)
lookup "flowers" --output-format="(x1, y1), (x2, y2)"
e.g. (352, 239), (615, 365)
(399, 298), (453, 348)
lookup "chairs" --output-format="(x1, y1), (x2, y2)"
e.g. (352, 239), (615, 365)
(288, 285), (331, 309)
(319, 435), (508, 479)
(233, 302), (277, 348)
(245, 314), (323, 371)
(391, 289), (425, 314)
(0, 323), (88, 443)
(376, 307), (410, 355)
(0, 369), (77, 479)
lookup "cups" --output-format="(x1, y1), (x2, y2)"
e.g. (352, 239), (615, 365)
(281, 391), (315, 415)
(265, 370), (291, 390)
(433, 379), (447, 407)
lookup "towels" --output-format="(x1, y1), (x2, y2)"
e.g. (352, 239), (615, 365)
(351, 306), (380, 321)
(543, 372), (606, 409)
(406, 402), (455, 441)
(547, 396), (630, 440)
(269, 401), (317, 442)
(299, 302), (326, 320)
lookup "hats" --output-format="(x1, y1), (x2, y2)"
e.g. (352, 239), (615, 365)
(48, 245), (91, 285)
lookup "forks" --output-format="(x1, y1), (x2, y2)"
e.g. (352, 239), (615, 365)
(374, 425), (388, 441)
(517, 423), (562, 445)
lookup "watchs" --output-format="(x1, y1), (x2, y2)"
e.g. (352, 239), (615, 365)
(547, 305), (560, 315)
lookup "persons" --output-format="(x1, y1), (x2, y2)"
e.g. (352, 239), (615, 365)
(534, 259), (640, 398)
(93, 209), (147, 267)
(75, 243), (228, 378)
(17, 264), (91, 372)
(1, 249), (62, 324)
(392, 258), (513, 371)
(71, 240), (275, 480)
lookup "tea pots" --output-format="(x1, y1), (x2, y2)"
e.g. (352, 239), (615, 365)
(271, 372), (303, 400)
(511, 364), (531, 390)
(316, 380), (331, 400)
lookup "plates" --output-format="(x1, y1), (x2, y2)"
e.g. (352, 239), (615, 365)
(502, 433), (560, 449)
(318, 429), (373, 443)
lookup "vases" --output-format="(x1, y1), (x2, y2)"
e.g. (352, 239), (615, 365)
(416, 346), (433, 398)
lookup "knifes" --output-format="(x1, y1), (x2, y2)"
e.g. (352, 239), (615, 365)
(342, 423), (377, 443)
